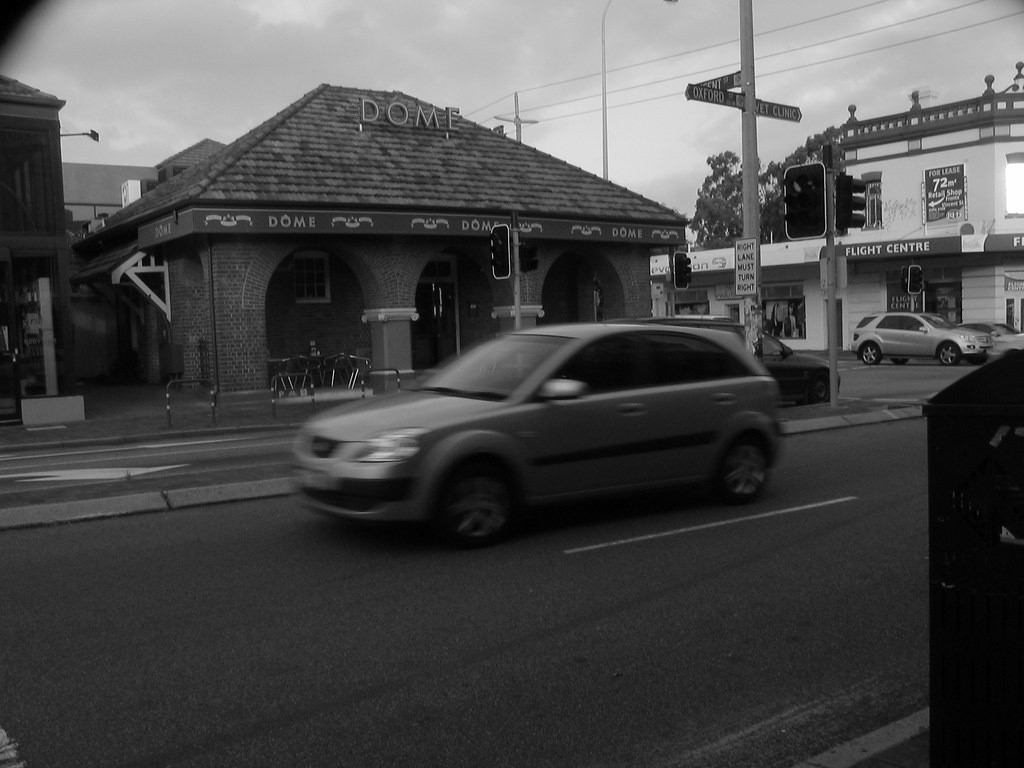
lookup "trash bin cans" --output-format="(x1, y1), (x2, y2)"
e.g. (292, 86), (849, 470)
(921, 349), (1024, 768)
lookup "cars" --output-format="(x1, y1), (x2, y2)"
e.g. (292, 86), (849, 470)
(957, 320), (1024, 358)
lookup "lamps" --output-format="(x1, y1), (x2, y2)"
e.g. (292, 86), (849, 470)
(60, 130), (99, 142)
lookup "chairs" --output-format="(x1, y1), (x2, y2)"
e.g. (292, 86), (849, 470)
(274, 352), (374, 393)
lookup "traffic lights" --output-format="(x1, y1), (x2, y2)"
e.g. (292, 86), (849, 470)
(673, 254), (693, 292)
(835, 171), (868, 230)
(781, 161), (828, 241)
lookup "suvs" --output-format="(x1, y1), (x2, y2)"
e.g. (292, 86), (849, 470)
(288, 314), (782, 548)
(850, 310), (995, 366)
